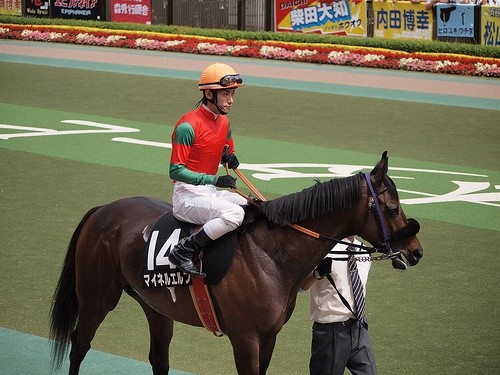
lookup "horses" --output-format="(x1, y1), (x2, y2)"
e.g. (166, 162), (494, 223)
(47, 150), (423, 375)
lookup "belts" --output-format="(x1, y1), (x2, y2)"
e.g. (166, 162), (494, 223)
(314, 319), (359, 327)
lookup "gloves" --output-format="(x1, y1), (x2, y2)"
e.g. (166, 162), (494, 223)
(222, 154), (240, 169)
(313, 257), (332, 279)
(216, 175), (238, 189)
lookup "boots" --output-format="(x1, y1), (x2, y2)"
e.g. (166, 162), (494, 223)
(168, 233), (207, 279)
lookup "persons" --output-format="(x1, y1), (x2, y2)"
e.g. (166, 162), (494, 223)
(301, 235), (378, 375)
(168, 62), (247, 277)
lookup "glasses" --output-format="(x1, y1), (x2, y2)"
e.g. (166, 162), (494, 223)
(219, 72), (243, 86)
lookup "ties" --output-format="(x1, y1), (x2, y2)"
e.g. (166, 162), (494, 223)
(346, 244), (366, 324)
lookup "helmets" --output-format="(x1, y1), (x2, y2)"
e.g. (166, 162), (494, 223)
(199, 63), (243, 91)
